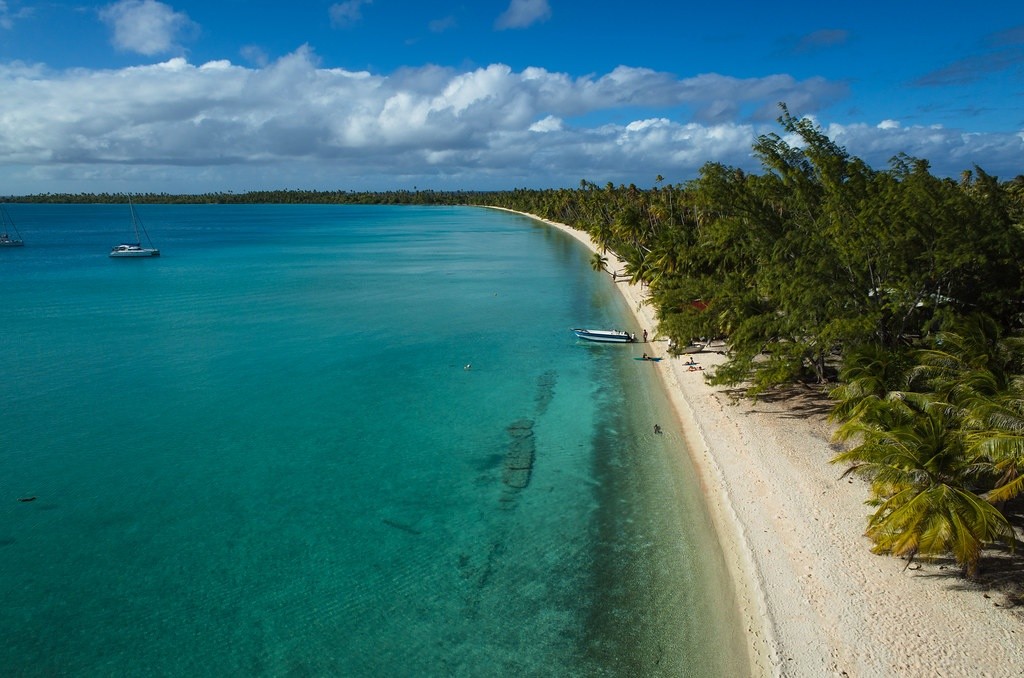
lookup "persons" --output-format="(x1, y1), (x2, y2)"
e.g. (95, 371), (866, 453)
(642, 353), (651, 360)
(689, 366), (701, 370)
(668, 337), (672, 349)
(642, 329), (648, 343)
(685, 356), (694, 364)
(630, 331), (637, 342)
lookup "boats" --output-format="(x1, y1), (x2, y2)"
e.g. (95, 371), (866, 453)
(110, 193), (160, 257)
(0, 201), (24, 247)
(570, 327), (633, 343)
